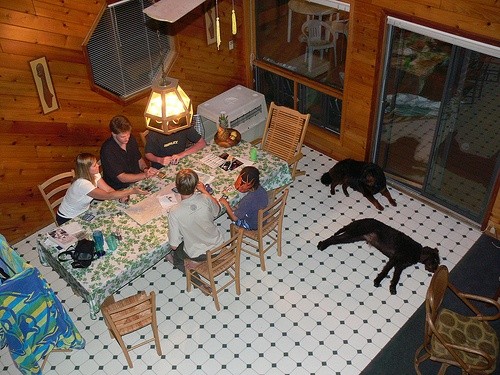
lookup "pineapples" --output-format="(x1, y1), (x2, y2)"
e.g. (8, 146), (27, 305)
(218, 113), (229, 141)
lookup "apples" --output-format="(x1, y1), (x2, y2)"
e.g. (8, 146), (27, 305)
(229, 131), (237, 141)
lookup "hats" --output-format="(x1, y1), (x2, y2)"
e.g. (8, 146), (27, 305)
(235, 166), (260, 193)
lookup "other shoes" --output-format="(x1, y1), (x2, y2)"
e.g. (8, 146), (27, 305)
(202, 287), (212, 296)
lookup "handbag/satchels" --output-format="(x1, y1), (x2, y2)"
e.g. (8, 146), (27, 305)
(57, 239), (95, 268)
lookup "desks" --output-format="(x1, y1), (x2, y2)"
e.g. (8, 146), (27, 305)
(390, 34), (451, 99)
(287, 0), (336, 43)
(284, 51), (332, 112)
(35, 138), (293, 320)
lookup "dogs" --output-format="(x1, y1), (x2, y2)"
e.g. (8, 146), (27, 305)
(435, 130), (500, 185)
(320, 159), (397, 211)
(379, 137), (427, 177)
(317, 218), (440, 295)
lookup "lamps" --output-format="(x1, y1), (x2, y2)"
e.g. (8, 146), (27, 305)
(140, 0), (194, 135)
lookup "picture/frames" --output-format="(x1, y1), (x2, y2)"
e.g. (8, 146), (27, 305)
(28, 55), (60, 116)
(204, 6), (217, 47)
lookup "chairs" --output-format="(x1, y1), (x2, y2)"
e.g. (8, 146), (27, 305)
(102, 290), (163, 370)
(37, 127), (152, 228)
(414, 265), (500, 375)
(441, 54), (495, 105)
(301, 19), (337, 73)
(230, 188), (289, 271)
(262, 57), (318, 115)
(248, 100), (312, 196)
(184, 222), (244, 312)
(319, 71), (345, 129)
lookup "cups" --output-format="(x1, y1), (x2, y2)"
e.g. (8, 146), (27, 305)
(105, 235), (117, 250)
(250, 148), (257, 160)
(93, 229), (104, 245)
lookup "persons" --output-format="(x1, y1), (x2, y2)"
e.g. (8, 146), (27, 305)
(100, 114), (158, 189)
(56, 153), (149, 227)
(145, 117), (206, 170)
(165, 168), (223, 295)
(219, 166), (268, 229)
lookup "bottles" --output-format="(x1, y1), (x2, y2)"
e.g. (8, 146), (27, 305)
(95, 241), (105, 256)
